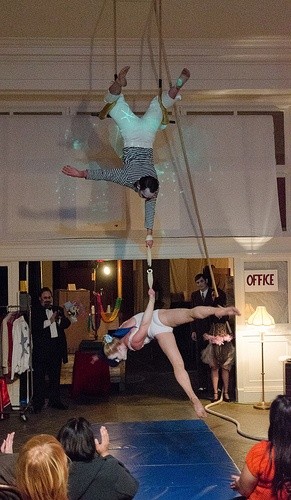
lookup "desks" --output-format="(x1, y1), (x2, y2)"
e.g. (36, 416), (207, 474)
(73, 339), (111, 396)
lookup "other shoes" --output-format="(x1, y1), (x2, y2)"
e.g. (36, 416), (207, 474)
(212, 393), (219, 402)
(51, 400), (67, 409)
(224, 393), (230, 402)
(35, 405), (42, 414)
(198, 386), (208, 393)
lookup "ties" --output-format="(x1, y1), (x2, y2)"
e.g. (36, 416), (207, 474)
(201, 293), (204, 302)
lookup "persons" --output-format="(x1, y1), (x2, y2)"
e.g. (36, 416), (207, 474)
(60, 65), (194, 248)
(103, 288), (242, 418)
(55, 417), (139, 500)
(0, 431), (22, 489)
(211, 307), (235, 402)
(229, 394), (291, 500)
(30, 287), (72, 412)
(16, 433), (70, 500)
(191, 274), (216, 342)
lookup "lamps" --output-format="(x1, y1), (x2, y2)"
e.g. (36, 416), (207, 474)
(248, 306), (276, 410)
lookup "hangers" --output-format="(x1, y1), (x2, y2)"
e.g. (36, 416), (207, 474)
(7, 305), (25, 317)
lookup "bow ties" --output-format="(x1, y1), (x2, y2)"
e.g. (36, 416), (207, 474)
(42, 306), (51, 310)
